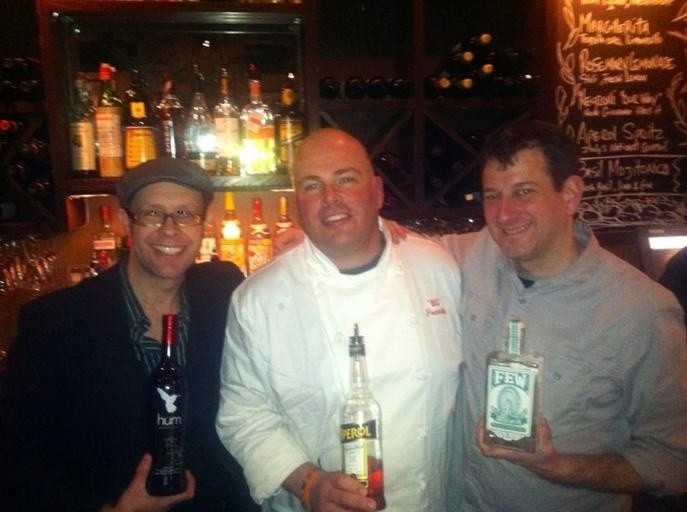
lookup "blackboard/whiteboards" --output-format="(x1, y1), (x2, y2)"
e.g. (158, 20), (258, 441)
(546, 0), (687, 228)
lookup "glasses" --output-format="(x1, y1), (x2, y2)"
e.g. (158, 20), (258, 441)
(127, 206), (204, 228)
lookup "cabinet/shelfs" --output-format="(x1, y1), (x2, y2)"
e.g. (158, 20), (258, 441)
(36, 4), (313, 196)
(1, 1), (88, 240)
(298, 0), (559, 239)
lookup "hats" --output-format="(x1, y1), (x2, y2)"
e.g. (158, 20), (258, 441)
(116, 157), (212, 210)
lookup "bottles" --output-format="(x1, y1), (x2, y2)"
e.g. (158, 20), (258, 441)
(430, 23), (499, 95)
(0, 50), (50, 223)
(317, 75), (411, 100)
(340, 321), (386, 510)
(91, 190), (290, 278)
(145, 310), (188, 495)
(369, 106), (512, 212)
(66, 59), (303, 176)
(480, 319), (542, 450)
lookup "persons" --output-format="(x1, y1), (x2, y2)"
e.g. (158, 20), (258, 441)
(382, 117), (687, 512)
(214, 126), (463, 512)
(13, 156), (263, 511)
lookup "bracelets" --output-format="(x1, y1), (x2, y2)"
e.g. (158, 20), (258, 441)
(300, 467), (323, 512)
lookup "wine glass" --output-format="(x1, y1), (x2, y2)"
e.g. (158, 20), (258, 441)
(0, 234), (58, 302)
